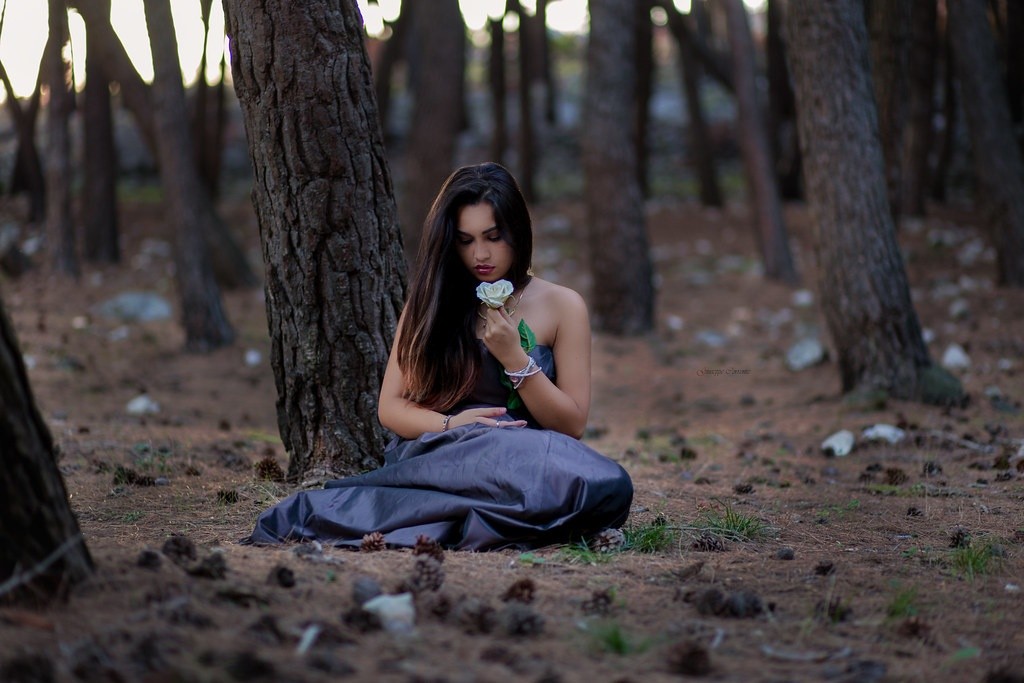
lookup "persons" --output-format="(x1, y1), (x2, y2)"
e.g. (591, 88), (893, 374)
(237, 162), (634, 545)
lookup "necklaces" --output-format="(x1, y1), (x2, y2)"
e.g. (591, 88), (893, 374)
(478, 285), (526, 327)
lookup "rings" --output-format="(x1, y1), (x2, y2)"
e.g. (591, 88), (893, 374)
(496, 419), (499, 427)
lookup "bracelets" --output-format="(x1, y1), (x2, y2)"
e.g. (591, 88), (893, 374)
(510, 359), (536, 383)
(441, 415), (452, 431)
(505, 356), (542, 389)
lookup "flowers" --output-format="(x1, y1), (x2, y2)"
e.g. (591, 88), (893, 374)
(475, 280), (537, 409)
